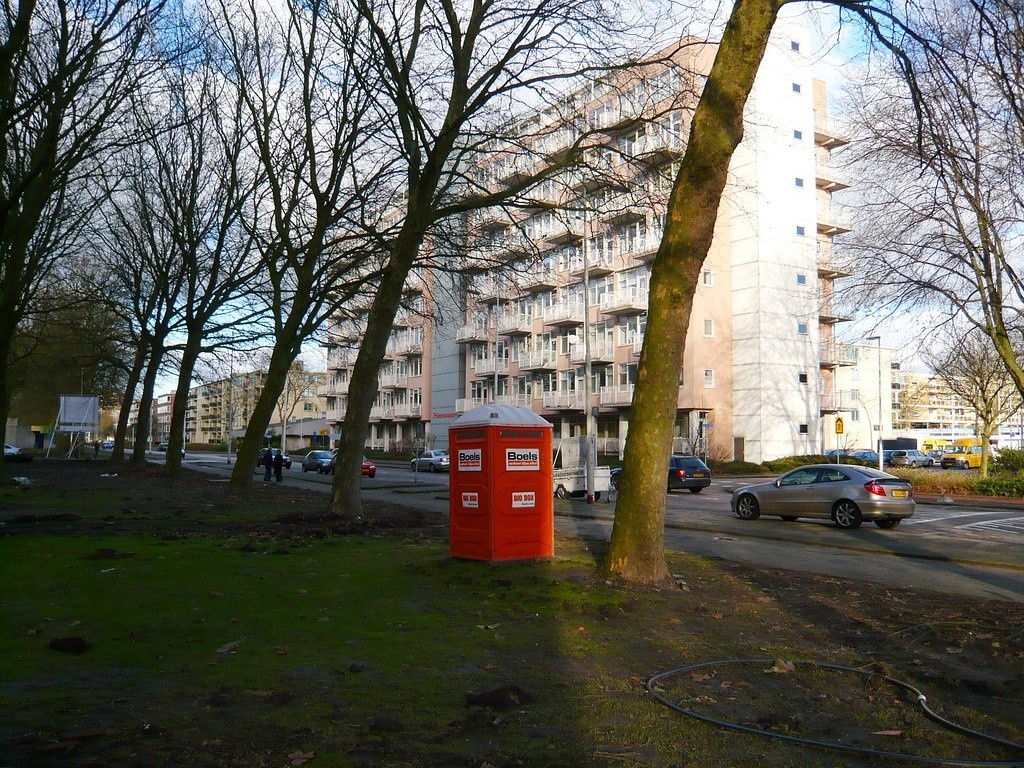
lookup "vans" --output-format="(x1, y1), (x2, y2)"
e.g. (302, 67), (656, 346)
(940, 438), (993, 470)
(920, 439), (949, 455)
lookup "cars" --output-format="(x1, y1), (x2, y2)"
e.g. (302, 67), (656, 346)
(301, 450), (335, 474)
(4, 444), (22, 456)
(825, 448), (934, 468)
(928, 449), (946, 459)
(411, 449), (451, 474)
(610, 454), (712, 494)
(256, 448), (292, 469)
(101, 440), (112, 449)
(157, 443), (168, 452)
(331, 450), (376, 479)
(165, 446), (186, 459)
(731, 463), (916, 531)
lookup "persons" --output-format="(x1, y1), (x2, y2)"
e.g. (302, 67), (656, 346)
(263, 447), (283, 482)
(94, 441), (100, 457)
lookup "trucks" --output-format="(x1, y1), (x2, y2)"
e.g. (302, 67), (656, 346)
(553, 466), (611, 501)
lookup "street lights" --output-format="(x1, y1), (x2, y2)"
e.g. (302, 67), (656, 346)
(586, 177), (631, 503)
(465, 287), (498, 402)
(227, 332), (257, 465)
(865, 336), (884, 471)
